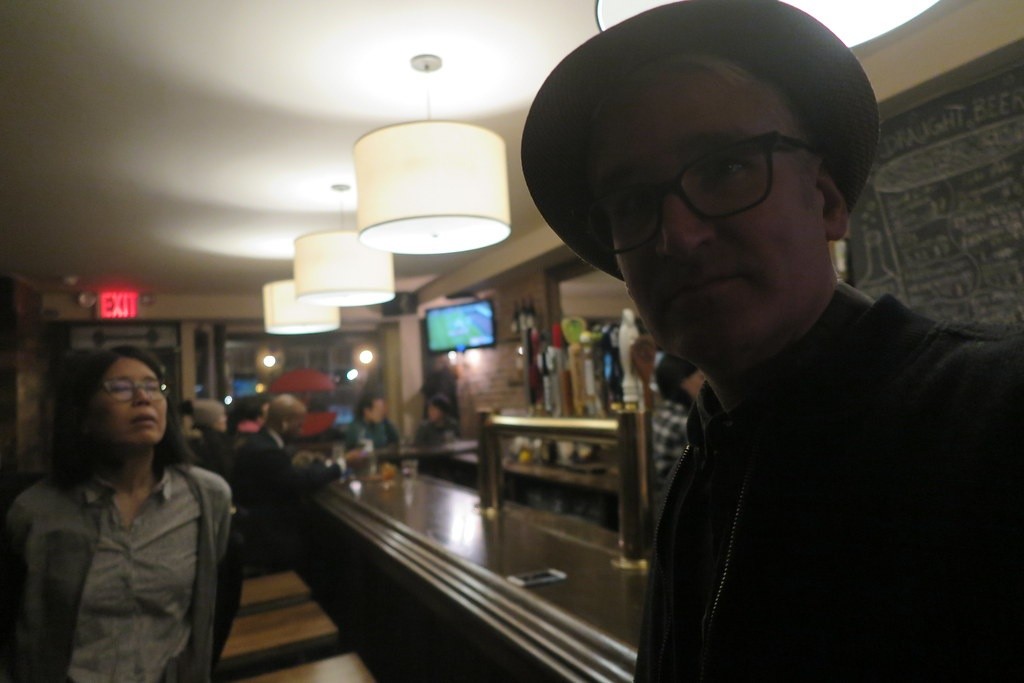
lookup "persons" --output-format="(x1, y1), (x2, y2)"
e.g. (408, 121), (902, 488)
(0, 344), (370, 683)
(413, 391), (478, 485)
(346, 397), (402, 477)
(650, 356), (703, 518)
(521, 0), (1024, 683)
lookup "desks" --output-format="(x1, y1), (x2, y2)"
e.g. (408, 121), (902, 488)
(345, 434), (479, 473)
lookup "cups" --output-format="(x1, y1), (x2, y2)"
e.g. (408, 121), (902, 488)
(333, 446), (347, 484)
(401, 460), (418, 480)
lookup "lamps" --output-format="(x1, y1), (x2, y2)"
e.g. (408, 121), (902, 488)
(77, 289), (155, 319)
(293, 183), (396, 308)
(353, 53), (513, 256)
(263, 278), (346, 336)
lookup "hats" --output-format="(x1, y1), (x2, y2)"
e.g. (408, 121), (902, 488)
(192, 400), (224, 423)
(521, 0), (880, 282)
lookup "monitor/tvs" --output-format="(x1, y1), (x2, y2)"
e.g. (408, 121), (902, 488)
(425, 297), (498, 355)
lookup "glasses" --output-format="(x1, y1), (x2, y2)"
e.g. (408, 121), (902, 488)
(102, 378), (167, 402)
(585, 131), (815, 254)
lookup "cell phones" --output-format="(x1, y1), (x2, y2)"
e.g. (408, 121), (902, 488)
(507, 569), (568, 587)
